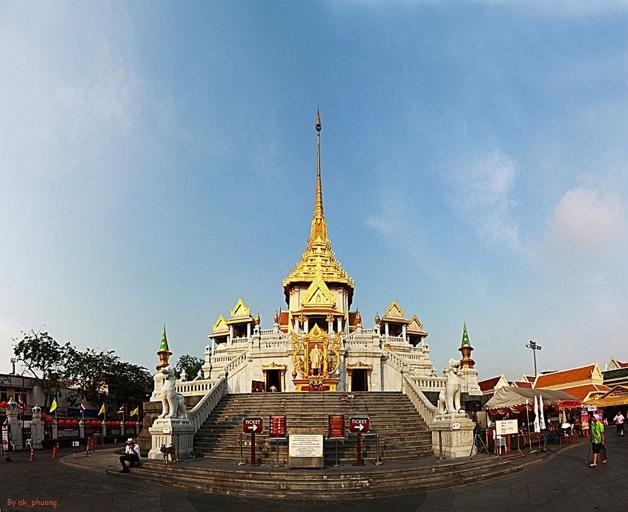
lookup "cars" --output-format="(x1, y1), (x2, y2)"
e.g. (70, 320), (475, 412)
(111, 429), (119, 435)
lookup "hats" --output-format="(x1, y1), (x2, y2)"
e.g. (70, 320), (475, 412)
(593, 414), (599, 422)
(125, 438), (135, 444)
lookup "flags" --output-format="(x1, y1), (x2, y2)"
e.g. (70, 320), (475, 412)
(79, 399), (89, 413)
(18, 394), (27, 410)
(129, 407), (138, 417)
(49, 397), (58, 413)
(98, 404), (105, 415)
(117, 405), (124, 414)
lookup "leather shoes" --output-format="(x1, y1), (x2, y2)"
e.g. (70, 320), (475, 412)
(590, 464), (597, 466)
(602, 459), (607, 464)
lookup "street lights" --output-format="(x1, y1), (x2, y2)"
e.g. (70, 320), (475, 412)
(526, 339), (542, 379)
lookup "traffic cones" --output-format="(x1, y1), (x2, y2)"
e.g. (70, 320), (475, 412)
(49, 446), (59, 458)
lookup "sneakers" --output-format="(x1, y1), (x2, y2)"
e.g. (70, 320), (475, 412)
(120, 469), (130, 473)
(130, 464), (133, 468)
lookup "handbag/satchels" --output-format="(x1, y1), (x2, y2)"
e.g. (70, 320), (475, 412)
(601, 439), (608, 459)
(133, 462), (139, 468)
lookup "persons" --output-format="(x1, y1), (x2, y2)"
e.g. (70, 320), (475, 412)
(270, 384), (278, 393)
(500, 408), (578, 441)
(589, 413), (608, 466)
(309, 343), (324, 376)
(612, 411), (625, 437)
(119, 437), (142, 472)
